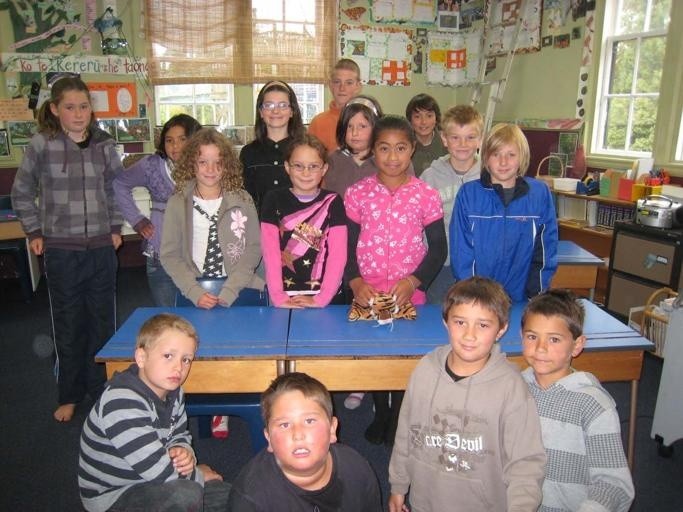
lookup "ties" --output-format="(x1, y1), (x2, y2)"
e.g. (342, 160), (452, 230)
(193, 201), (223, 279)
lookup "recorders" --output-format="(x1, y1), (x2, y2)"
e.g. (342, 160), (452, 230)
(631, 194), (683, 228)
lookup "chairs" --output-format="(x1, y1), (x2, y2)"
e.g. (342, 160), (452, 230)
(285, 298), (655, 472)
(95, 305), (289, 458)
(546, 239), (604, 301)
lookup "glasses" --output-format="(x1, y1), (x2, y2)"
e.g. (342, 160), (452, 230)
(262, 103), (289, 109)
(291, 163), (324, 173)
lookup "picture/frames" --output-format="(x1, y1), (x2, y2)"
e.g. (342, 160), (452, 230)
(557, 132), (578, 168)
(0, 103), (151, 157)
(437, 10), (460, 33)
(219, 126), (247, 150)
(548, 152), (568, 178)
(542, 36), (552, 47)
(346, 40), (367, 56)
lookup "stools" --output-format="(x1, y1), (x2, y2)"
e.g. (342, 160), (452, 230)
(0, 222), (40, 291)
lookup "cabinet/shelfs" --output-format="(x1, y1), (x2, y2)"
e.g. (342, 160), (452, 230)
(556, 191), (636, 294)
(523, 129), (559, 178)
(605, 218), (682, 328)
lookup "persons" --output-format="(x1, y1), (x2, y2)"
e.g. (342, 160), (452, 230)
(241, 80), (348, 308)
(77, 313), (231, 511)
(449, 122), (559, 304)
(519, 285), (635, 512)
(309, 59), (448, 445)
(228, 372), (384, 512)
(407, 94), (482, 303)
(10, 78), (125, 423)
(113, 113), (263, 441)
(387, 277), (547, 512)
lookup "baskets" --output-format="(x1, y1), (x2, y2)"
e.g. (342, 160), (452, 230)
(536, 156), (565, 191)
